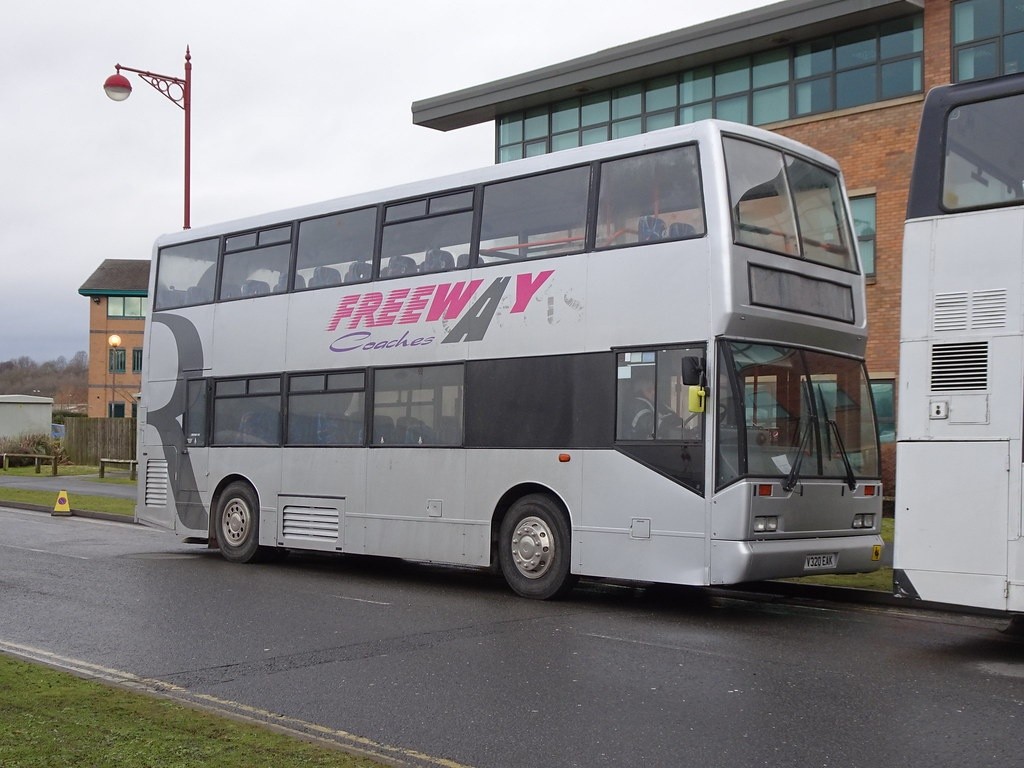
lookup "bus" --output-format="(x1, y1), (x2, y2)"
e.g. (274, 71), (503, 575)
(134, 118), (885, 600)
(894, 71), (1023, 641)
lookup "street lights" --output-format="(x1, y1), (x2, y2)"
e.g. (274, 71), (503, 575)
(110, 335), (122, 417)
(104, 43), (192, 231)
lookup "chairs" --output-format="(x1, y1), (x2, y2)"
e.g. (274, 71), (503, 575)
(242, 412), (461, 445)
(639, 216), (695, 242)
(223, 248), (485, 301)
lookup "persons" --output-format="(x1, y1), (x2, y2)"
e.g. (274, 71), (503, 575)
(623, 376), (681, 439)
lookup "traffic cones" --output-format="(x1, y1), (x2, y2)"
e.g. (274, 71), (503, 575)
(52, 490), (73, 518)
